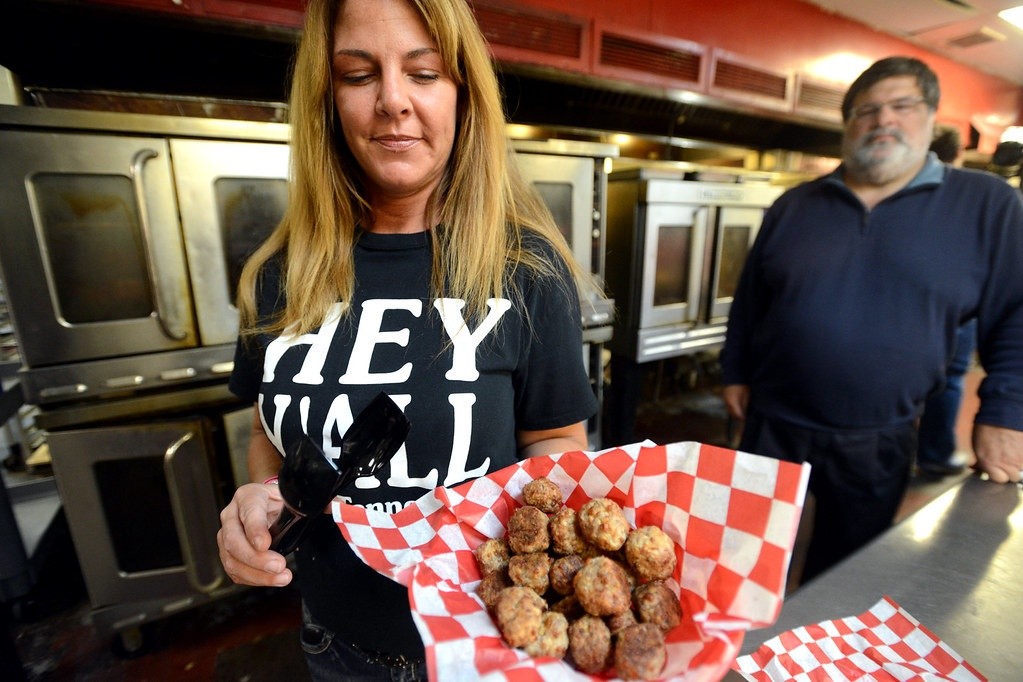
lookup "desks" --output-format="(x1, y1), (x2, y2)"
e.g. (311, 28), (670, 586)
(710, 476), (1023, 680)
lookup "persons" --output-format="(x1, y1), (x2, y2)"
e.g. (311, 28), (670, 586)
(217, 0), (591, 682)
(719, 56), (1023, 590)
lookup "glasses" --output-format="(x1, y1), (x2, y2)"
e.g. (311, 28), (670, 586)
(846, 98), (928, 122)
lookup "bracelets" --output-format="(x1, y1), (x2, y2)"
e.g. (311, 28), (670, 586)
(263, 476), (279, 485)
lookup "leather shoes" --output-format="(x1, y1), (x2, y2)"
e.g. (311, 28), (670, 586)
(915, 455), (970, 484)
